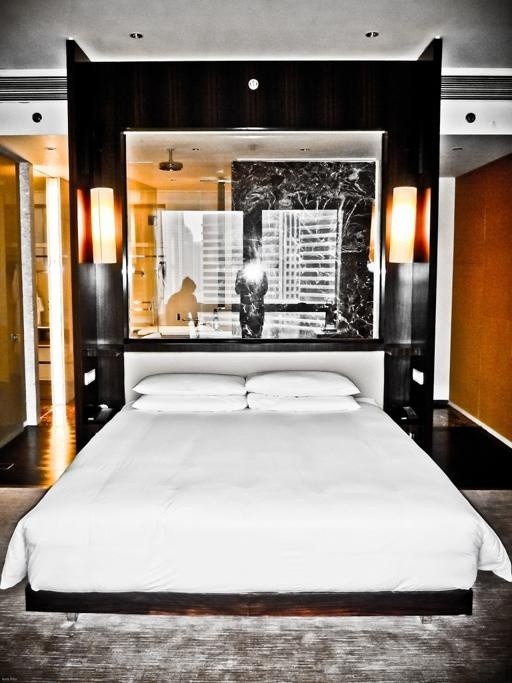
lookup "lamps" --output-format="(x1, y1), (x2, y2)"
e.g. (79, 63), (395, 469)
(90, 186), (117, 264)
(386, 185), (419, 264)
(157, 146), (183, 172)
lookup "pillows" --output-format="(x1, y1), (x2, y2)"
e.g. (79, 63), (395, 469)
(249, 395), (366, 410)
(245, 369), (363, 394)
(130, 371), (246, 395)
(133, 396), (247, 411)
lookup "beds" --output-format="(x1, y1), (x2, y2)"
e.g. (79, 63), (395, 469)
(0, 347), (512, 625)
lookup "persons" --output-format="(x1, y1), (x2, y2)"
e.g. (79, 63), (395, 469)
(234, 252), (268, 341)
(166, 275), (200, 338)
